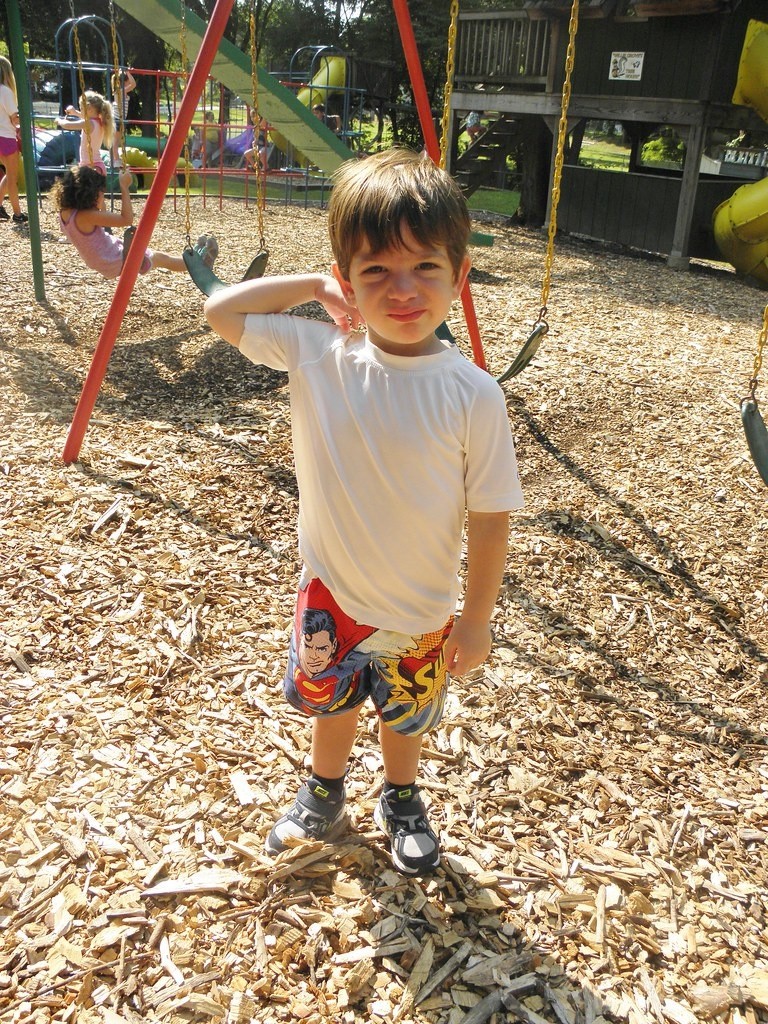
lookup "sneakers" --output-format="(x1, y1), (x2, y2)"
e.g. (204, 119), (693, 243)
(374, 793), (443, 876)
(198, 239), (218, 270)
(264, 778), (346, 855)
(194, 235), (206, 251)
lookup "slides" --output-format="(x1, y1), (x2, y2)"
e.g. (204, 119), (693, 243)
(709, 19), (768, 282)
(113, 0), (496, 246)
(266, 57), (346, 169)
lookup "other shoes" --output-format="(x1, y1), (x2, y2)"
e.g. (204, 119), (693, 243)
(0, 207), (10, 218)
(13, 213), (28, 222)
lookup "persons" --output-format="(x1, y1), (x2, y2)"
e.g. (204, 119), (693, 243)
(201, 111), (221, 167)
(312, 103), (341, 138)
(54, 166), (218, 280)
(244, 107), (271, 172)
(53, 89), (117, 234)
(198, 148), (526, 877)
(108, 68), (137, 168)
(192, 126), (203, 159)
(0, 56), (31, 222)
(465, 107), (487, 146)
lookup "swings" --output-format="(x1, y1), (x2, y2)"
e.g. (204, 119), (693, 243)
(740, 305), (768, 486)
(68, 0), (138, 279)
(429, 0), (580, 384)
(178, 0), (271, 297)
